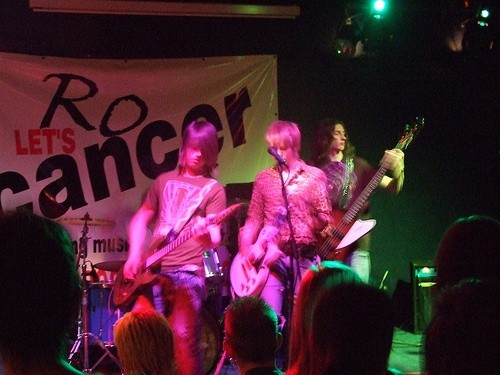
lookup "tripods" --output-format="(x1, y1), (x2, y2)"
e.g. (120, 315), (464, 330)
(67, 212), (121, 374)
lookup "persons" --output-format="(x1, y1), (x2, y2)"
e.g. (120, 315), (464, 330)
(223, 295), (285, 375)
(285, 261), (402, 375)
(312, 118), (405, 285)
(122, 120), (227, 375)
(238, 120), (336, 375)
(113, 308), (174, 375)
(0, 210), (86, 375)
(421, 215), (500, 375)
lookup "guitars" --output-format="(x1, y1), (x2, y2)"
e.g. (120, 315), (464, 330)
(229, 137), (342, 298)
(112, 203), (241, 308)
(317, 124), (416, 261)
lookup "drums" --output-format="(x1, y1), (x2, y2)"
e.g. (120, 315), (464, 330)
(78, 280), (132, 348)
(163, 308), (224, 375)
(204, 244), (230, 278)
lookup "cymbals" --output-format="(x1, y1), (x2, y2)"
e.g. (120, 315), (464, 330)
(68, 218), (119, 228)
(93, 260), (126, 272)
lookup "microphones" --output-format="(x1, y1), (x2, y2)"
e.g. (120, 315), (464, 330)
(90, 262), (100, 289)
(267, 146), (289, 168)
